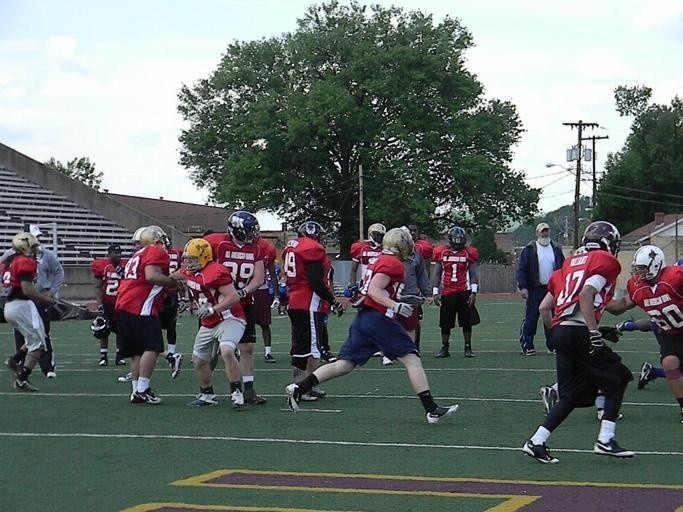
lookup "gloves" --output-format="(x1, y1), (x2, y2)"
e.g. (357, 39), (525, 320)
(543, 323), (555, 352)
(599, 325), (623, 343)
(348, 284), (358, 297)
(235, 288), (249, 300)
(269, 296), (280, 312)
(392, 302), (415, 318)
(195, 306), (215, 320)
(97, 304), (105, 315)
(586, 329), (607, 363)
(330, 298), (344, 317)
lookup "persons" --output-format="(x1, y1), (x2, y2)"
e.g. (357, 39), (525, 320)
(164, 234), (182, 379)
(516, 222), (565, 356)
(281, 221), (344, 402)
(115, 225), (179, 406)
(254, 251), (287, 364)
(382, 226), (433, 366)
(116, 226), (148, 384)
(615, 315), (665, 390)
(0, 223), (64, 379)
(605, 245), (683, 424)
(673, 259), (683, 266)
(314, 256), (338, 365)
(521, 221), (635, 465)
(406, 222), (433, 351)
(350, 223), (386, 357)
(0, 232), (57, 392)
(539, 246), (624, 421)
(431, 226), (481, 359)
(285, 228), (459, 425)
(163, 238), (248, 410)
(266, 262), (286, 316)
(202, 211), (266, 405)
(92, 243), (129, 366)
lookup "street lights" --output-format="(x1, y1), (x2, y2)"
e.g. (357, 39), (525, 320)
(566, 142), (592, 250)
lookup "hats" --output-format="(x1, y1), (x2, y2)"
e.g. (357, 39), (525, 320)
(23, 222), (43, 236)
(107, 244), (125, 251)
(535, 222), (552, 232)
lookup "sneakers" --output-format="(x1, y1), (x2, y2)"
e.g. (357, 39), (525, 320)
(537, 385), (558, 419)
(300, 392), (319, 401)
(616, 319), (632, 336)
(592, 437), (635, 459)
(545, 347), (557, 355)
(319, 346), (338, 363)
(264, 353), (278, 363)
(185, 394), (219, 408)
(230, 388), (245, 408)
(46, 370), (56, 378)
(12, 377), (40, 391)
(381, 356), (395, 366)
(424, 403), (459, 425)
(372, 352), (382, 357)
(596, 410), (624, 422)
(520, 438), (561, 465)
(434, 350), (451, 358)
(525, 347), (537, 357)
(129, 388), (163, 405)
(114, 355), (126, 366)
(243, 390), (267, 405)
(117, 371), (133, 383)
(285, 383), (302, 414)
(4, 356), (24, 378)
(168, 353), (184, 379)
(307, 390), (326, 398)
(637, 360), (654, 391)
(464, 347), (475, 358)
(97, 352), (109, 368)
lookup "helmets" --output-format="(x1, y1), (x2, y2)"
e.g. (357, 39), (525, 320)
(631, 244), (668, 281)
(226, 210), (261, 244)
(132, 226), (147, 242)
(89, 315), (111, 340)
(181, 237), (214, 271)
(447, 225), (466, 251)
(11, 232), (40, 256)
(381, 227), (415, 262)
(139, 225), (168, 247)
(368, 222), (387, 242)
(581, 220), (622, 259)
(297, 221), (327, 243)
